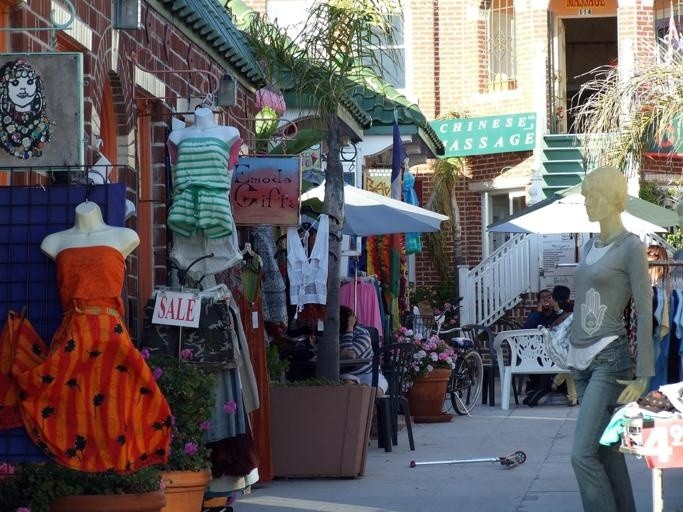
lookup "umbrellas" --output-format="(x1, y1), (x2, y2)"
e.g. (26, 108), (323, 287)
(301, 176), (450, 321)
(486, 179), (683, 238)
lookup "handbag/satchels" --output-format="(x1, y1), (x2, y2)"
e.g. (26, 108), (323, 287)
(538, 314), (572, 369)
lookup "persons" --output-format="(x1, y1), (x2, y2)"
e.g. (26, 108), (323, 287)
(569, 167), (655, 512)
(521, 289), (567, 407)
(1, 203), (174, 475)
(0, 59), (56, 158)
(286, 334), (322, 381)
(340, 303), (390, 447)
(401, 158), (423, 253)
(168, 108), (240, 240)
(553, 285), (574, 312)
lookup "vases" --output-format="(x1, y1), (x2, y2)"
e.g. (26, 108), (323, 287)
(405, 367), (453, 423)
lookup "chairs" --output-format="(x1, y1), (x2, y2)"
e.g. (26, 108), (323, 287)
(462, 324), (519, 406)
(358, 326), (416, 452)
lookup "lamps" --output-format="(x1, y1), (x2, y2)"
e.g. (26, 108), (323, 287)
(216, 73), (237, 108)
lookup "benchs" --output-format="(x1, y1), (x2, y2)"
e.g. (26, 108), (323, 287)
(493, 328), (573, 410)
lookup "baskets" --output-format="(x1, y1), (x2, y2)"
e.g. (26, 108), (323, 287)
(402, 314), (432, 341)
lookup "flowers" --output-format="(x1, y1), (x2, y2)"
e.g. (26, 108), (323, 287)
(0, 347), (237, 512)
(379, 326), (457, 392)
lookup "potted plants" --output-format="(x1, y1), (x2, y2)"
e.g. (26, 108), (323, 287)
(265, 344), (375, 485)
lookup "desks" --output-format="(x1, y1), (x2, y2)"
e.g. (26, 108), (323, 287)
(308, 358), (372, 379)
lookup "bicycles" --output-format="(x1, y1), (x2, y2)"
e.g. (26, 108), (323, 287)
(401, 296), (484, 418)
